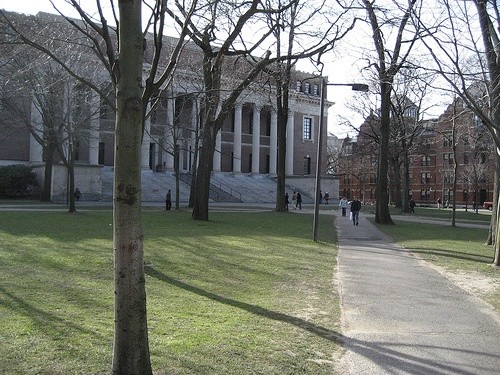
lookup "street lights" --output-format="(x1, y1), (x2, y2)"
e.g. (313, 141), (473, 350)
(312, 77), (369, 242)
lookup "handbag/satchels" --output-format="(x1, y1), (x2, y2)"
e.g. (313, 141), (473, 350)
(349, 212), (353, 220)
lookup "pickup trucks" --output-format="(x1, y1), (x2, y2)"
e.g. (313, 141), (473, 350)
(483, 202), (494, 211)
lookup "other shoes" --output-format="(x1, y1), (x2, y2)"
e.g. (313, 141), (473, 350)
(356, 223), (358, 225)
(353, 223), (355, 225)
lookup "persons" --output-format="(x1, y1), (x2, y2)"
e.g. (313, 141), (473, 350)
(339, 194), (348, 217)
(285, 193), (290, 210)
(165, 190), (171, 211)
(351, 196), (361, 226)
(410, 198), (417, 213)
(472, 202), (476, 211)
(75, 188), (80, 200)
(319, 190), (329, 204)
(292, 191), (303, 210)
(437, 198), (441, 209)
(342, 188), (352, 204)
